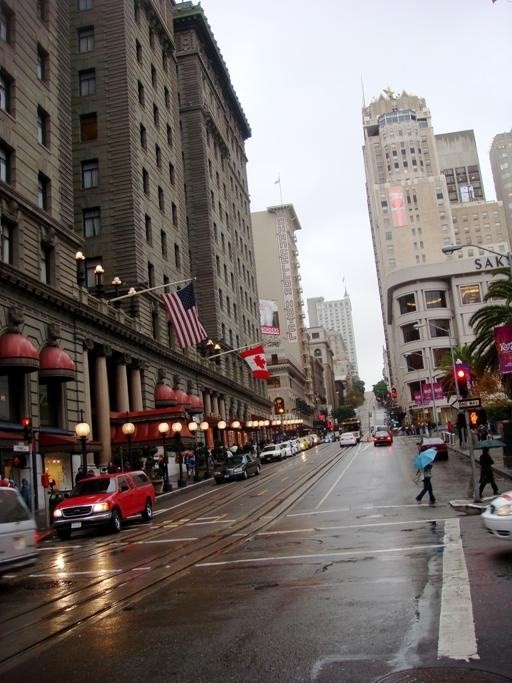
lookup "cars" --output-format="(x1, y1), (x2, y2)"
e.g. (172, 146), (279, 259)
(480, 490), (512, 540)
(416, 437), (448, 460)
(370, 425), (393, 446)
(213, 430), (339, 484)
(52, 470), (155, 540)
(339, 430), (361, 447)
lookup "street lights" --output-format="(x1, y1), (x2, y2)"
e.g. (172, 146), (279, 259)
(75, 410), (90, 472)
(400, 324), (460, 423)
(121, 411), (135, 465)
(441, 244), (512, 272)
(172, 419), (210, 487)
(157, 422), (172, 491)
(217, 419), (303, 452)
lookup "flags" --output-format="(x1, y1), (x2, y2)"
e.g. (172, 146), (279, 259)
(160, 280), (208, 349)
(239, 344), (269, 381)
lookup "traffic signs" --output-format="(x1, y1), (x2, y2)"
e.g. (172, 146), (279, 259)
(450, 398), (481, 411)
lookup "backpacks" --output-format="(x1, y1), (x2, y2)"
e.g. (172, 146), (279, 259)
(415, 467), (424, 481)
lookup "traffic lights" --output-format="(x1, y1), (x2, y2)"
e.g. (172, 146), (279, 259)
(274, 397), (285, 415)
(455, 359), (471, 398)
(469, 409), (480, 428)
(22, 418), (32, 445)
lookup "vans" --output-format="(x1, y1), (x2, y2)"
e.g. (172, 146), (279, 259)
(0, 486), (38, 575)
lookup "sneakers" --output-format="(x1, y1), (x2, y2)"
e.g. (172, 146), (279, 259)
(415, 498), (439, 504)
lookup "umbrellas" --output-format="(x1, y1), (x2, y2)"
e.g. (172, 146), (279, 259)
(473, 439), (505, 449)
(414, 448), (438, 469)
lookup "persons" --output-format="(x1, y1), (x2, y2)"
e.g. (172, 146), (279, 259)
(415, 460), (436, 504)
(76, 453), (195, 487)
(479, 447), (501, 498)
(405, 421), (429, 435)
(448, 421), (497, 444)
(207, 439), (281, 472)
